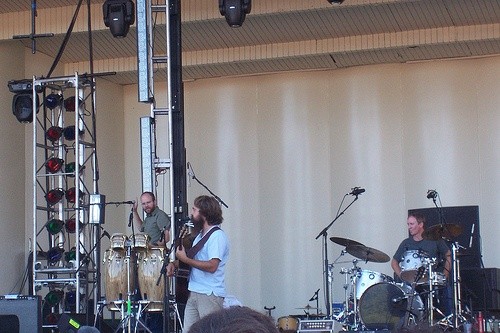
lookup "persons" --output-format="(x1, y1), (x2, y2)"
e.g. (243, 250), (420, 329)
(391, 215), (455, 318)
(188, 305), (276, 333)
(166, 196), (229, 333)
(133, 192), (171, 247)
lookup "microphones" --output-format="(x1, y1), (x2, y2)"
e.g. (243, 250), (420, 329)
(469, 223), (475, 247)
(128, 209), (133, 227)
(392, 298), (400, 302)
(178, 216), (192, 221)
(347, 189), (366, 195)
(187, 163), (191, 185)
(427, 191), (436, 199)
(159, 226), (166, 242)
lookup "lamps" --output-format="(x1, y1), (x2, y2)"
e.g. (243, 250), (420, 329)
(102, 0), (135, 38)
(219, 0), (251, 28)
(12, 92), (85, 310)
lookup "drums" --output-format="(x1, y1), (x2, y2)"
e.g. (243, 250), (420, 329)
(277, 315), (300, 332)
(356, 280), (426, 332)
(398, 252), (432, 282)
(136, 246), (165, 313)
(105, 245), (136, 312)
(354, 269), (394, 300)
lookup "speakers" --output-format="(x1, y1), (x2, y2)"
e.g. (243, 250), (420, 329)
(407, 205), (500, 311)
(56, 313), (116, 333)
(0, 294), (42, 333)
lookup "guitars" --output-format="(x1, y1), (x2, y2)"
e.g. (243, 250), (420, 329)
(172, 221), (194, 280)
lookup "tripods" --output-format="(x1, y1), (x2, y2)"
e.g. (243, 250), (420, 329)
(418, 233), (469, 333)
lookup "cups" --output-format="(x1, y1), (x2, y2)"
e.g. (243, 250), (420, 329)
(463, 323), (472, 333)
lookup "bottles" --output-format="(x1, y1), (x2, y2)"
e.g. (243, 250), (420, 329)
(474, 311), (484, 333)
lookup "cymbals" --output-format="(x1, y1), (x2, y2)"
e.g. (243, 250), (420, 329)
(294, 305), (320, 310)
(330, 236), (391, 263)
(422, 222), (465, 242)
